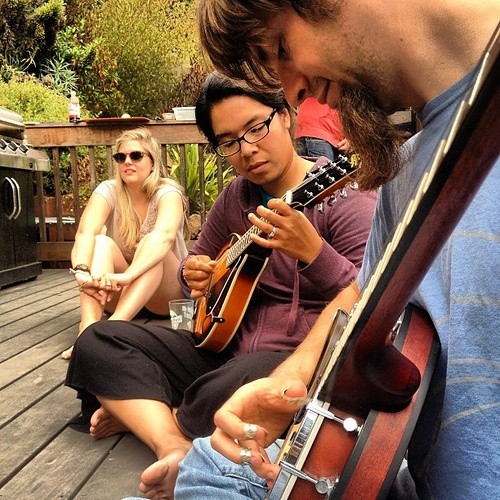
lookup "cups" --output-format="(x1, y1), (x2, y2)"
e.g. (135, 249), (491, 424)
(168, 298), (194, 333)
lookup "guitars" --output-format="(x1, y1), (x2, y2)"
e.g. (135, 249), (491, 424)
(193, 153), (363, 360)
(267, 19), (500, 500)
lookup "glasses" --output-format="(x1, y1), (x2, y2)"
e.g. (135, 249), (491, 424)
(113, 151), (151, 163)
(209, 107), (277, 159)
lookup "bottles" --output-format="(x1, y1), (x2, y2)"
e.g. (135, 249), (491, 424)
(68, 91), (80, 123)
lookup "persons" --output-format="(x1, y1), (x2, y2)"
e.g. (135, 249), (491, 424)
(65, 69), (380, 500)
(293, 96), (355, 162)
(59, 127), (189, 360)
(173, 0), (500, 500)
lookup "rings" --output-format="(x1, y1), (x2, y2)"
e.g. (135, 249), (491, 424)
(105, 281), (112, 286)
(267, 225), (278, 238)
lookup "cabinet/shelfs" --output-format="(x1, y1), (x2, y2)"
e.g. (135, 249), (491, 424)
(0, 166), (43, 289)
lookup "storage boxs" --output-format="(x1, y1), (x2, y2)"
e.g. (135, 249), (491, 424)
(172, 107), (196, 121)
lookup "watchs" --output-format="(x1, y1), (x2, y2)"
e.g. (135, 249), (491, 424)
(68, 264), (91, 274)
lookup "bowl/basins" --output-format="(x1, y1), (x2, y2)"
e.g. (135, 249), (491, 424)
(162, 112), (175, 120)
(172, 106), (196, 121)
